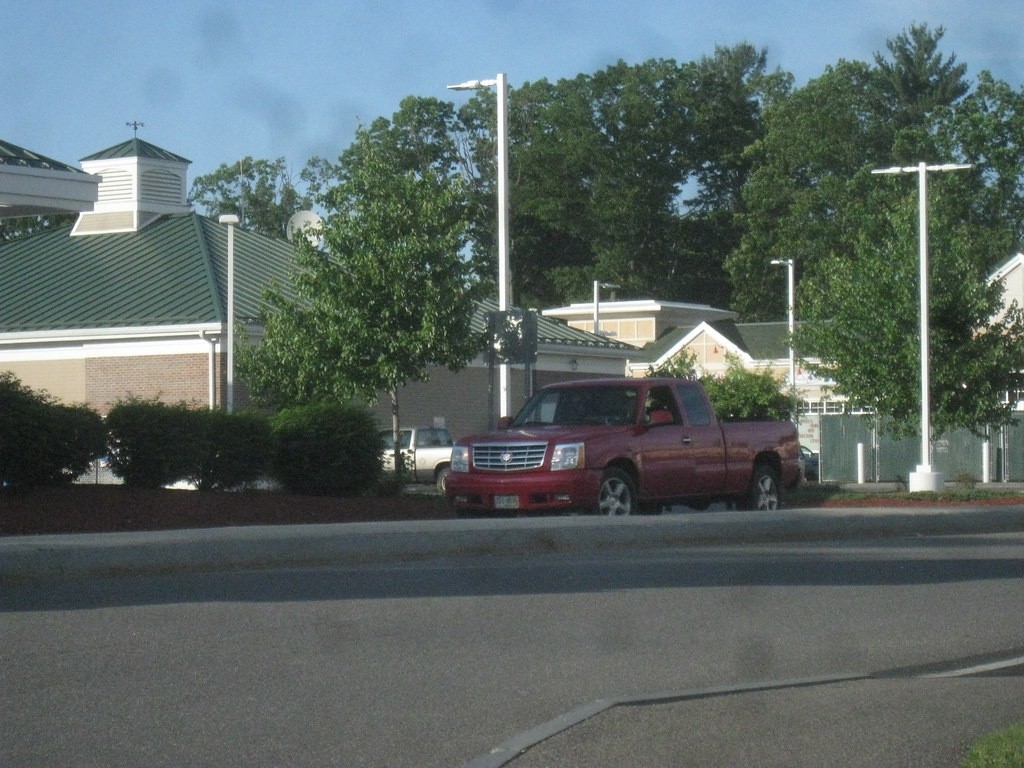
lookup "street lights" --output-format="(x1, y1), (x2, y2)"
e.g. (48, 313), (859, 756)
(593, 279), (621, 337)
(769, 256), (796, 428)
(867, 161), (977, 497)
(444, 71), (511, 423)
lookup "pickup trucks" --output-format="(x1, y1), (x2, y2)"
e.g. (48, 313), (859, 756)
(372, 427), (456, 495)
(442, 376), (802, 520)
